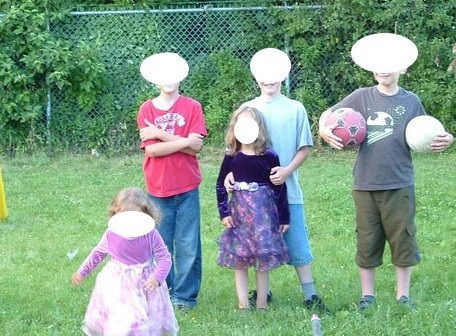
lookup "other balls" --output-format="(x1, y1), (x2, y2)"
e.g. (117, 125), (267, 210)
(405, 115), (445, 154)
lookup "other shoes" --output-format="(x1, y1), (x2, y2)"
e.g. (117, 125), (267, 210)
(397, 296), (410, 306)
(248, 289), (272, 307)
(359, 296), (375, 312)
(303, 294), (329, 314)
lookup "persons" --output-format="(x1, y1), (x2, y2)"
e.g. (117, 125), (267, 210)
(319, 33), (453, 312)
(137, 52), (208, 309)
(216, 48), (337, 315)
(72, 187), (179, 336)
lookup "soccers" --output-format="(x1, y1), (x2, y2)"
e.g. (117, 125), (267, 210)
(326, 107), (367, 149)
